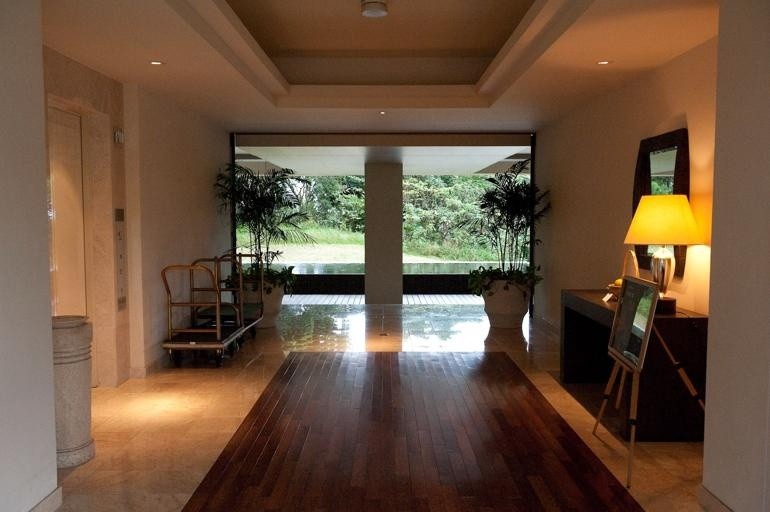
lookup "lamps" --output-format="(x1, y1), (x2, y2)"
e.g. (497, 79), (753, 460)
(623, 192), (708, 315)
(360, 1), (388, 17)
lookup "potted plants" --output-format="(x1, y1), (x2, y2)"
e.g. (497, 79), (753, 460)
(210, 160), (317, 330)
(465, 156), (553, 329)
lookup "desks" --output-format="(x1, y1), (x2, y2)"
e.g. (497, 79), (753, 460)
(558, 289), (708, 442)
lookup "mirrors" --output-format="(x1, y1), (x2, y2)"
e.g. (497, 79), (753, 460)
(632, 128), (690, 279)
